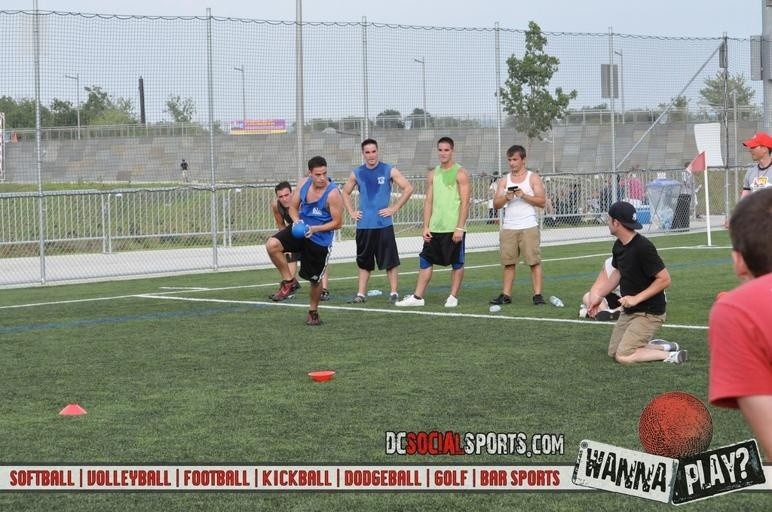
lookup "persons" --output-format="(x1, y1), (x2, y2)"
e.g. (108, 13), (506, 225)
(180, 159), (190, 182)
(394, 137), (471, 307)
(269, 182), (330, 301)
(488, 168), (706, 234)
(742, 132), (771, 200)
(339, 137), (413, 306)
(707, 186), (771, 464)
(585, 201), (689, 367)
(266, 155), (345, 326)
(577, 255), (623, 321)
(488, 145), (546, 305)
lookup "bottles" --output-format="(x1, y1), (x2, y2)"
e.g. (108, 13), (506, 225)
(549, 295), (564, 308)
(367, 290), (383, 297)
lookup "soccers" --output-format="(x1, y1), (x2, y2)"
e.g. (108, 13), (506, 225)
(292, 223), (309, 238)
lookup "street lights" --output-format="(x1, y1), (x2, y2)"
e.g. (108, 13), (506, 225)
(414, 55), (427, 129)
(64, 73), (81, 139)
(614, 48), (625, 126)
(234, 64), (246, 130)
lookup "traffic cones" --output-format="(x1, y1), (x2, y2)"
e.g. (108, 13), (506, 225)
(12, 133), (18, 143)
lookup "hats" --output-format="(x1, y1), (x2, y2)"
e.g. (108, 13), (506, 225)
(742, 131), (772, 151)
(607, 200), (645, 230)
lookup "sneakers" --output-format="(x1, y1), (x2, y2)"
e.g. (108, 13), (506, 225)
(668, 348), (689, 363)
(647, 338), (679, 351)
(579, 308), (589, 317)
(268, 278), (301, 302)
(595, 310), (622, 322)
(319, 289), (331, 301)
(394, 293), (426, 307)
(307, 309), (320, 325)
(488, 294), (512, 304)
(387, 293), (399, 304)
(346, 294), (366, 304)
(532, 294), (545, 305)
(443, 295), (459, 308)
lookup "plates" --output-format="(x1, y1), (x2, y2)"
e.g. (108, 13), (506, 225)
(59, 404), (87, 415)
(308, 371), (335, 381)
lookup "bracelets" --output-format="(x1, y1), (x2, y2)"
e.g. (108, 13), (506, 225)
(594, 294), (604, 299)
(455, 228), (466, 232)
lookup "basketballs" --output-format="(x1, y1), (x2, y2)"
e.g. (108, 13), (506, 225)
(639, 391), (713, 457)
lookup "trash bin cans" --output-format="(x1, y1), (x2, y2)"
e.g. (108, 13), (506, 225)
(646, 179), (683, 232)
